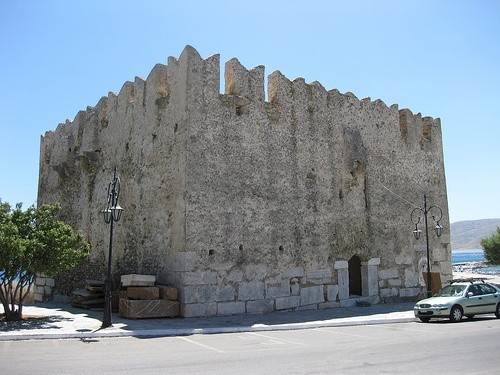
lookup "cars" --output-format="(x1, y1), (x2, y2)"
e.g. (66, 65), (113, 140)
(414, 277), (500, 322)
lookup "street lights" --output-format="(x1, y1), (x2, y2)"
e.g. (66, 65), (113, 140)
(100, 165), (124, 327)
(411, 194), (444, 299)
